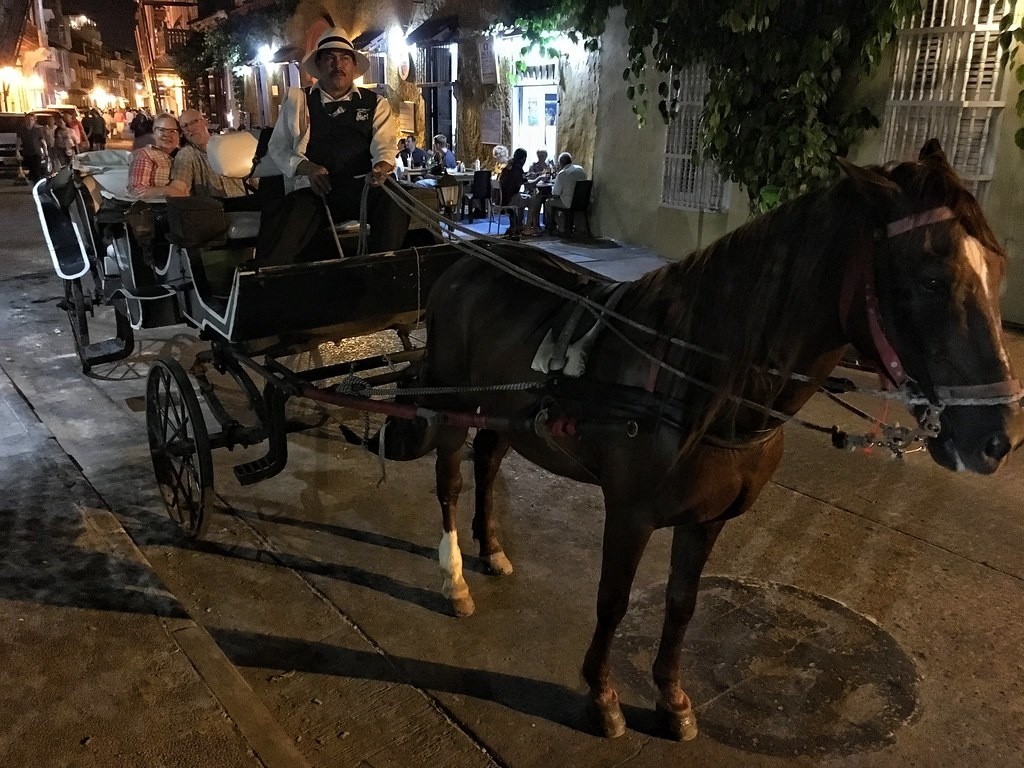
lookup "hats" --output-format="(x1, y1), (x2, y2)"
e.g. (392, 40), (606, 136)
(301, 27), (370, 80)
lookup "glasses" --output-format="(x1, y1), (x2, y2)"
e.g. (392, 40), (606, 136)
(181, 118), (202, 129)
(154, 127), (179, 134)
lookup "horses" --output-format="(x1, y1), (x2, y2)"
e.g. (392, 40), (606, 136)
(386, 137), (1024, 744)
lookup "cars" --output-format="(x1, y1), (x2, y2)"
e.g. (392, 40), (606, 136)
(0, 106), (83, 176)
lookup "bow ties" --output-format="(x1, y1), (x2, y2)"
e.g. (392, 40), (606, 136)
(324, 100), (356, 114)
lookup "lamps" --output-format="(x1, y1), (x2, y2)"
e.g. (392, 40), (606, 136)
(437, 169), (460, 242)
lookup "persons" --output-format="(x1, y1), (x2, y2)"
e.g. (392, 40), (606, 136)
(268, 27), (410, 264)
(395, 134), (456, 180)
(472, 145), (587, 240)
(16, 109), (260, 242)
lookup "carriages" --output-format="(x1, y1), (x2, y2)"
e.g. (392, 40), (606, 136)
(30, 128), (1024, 746)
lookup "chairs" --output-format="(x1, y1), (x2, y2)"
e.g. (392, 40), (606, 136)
(488, 180), (519, 235)
(461, 170), (492, 224)
(550, 179), (593, 237)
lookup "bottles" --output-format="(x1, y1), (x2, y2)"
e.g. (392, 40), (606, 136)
(422, 155), (435, 168)
(47, 157), (53, 172)
(474, 158), (480, 171)
(541, 168), (556, 180)
(460, 162), (466, 173)
(397, 153), (404, 172)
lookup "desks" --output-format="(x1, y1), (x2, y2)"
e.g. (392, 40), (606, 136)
(526, 178), (556, 203)
(404, 166), (431, 181)
(524, 179), (554, 235)
(447, 171), (475, 218)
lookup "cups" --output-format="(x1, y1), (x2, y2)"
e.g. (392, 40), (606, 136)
(407, 157), (414, 169)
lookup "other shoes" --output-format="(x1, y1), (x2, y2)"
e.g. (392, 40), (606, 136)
(524, 226), (534, 235)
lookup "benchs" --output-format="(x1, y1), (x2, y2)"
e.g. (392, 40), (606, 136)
(75, 129), (374, 297)
